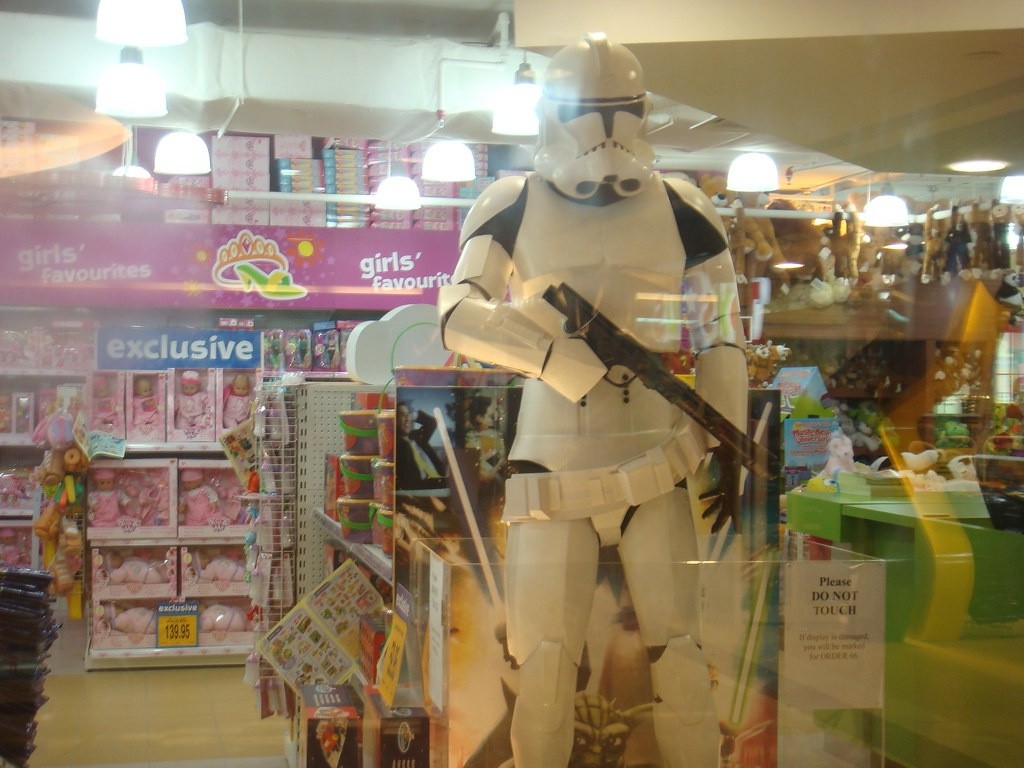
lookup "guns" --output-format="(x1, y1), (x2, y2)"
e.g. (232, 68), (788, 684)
(540, 281), (782, 483)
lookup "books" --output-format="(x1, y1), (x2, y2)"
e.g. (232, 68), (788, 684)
(69, 411), (127, 462)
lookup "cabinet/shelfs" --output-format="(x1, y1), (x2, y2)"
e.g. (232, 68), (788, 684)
(88, 367), (257, 667)
(0, 303), (94, 580)
(310, 510), (430, 720)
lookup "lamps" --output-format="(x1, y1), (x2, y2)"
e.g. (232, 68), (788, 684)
(97, 0), (1023, 228)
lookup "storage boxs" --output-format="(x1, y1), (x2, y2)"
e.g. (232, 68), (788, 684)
(298, 685), (430, 768)
(1, 314), (264, 646)
(3, 119), (491, 230)
(769, 367), (841, 469)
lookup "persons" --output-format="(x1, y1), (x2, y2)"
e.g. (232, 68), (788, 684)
(437, 31), (753, 768)
(178, 469), (218, 526)
(102, 603), (157, 634)
(396, 399), (462, 530)
(92, 376), (119, 426)
(99, 549), (170, 584)
(196, 600), (249, 633)
(174, 370), (210, 430)
(133, 378), (159, 428)
(0, 474), (18, 507)
(87, 469), (132, 528)
(466, 395), (509, 480)
(0, 528), (20, 566)
(189, 549), (246, 581)
(217, 486), (242, 500)
(270, 330), (336, 370)
(222, 372), (252, 429)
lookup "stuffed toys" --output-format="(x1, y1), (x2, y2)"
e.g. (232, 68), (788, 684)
(825, 342), (1024, 460)
(743, 337), (794, 388)
(698, 173), (1024, 326)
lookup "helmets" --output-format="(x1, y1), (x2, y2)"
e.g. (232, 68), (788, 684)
(530, 34), (653, 199)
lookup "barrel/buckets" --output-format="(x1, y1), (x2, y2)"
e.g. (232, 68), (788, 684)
(339, 408), (394, 552)
